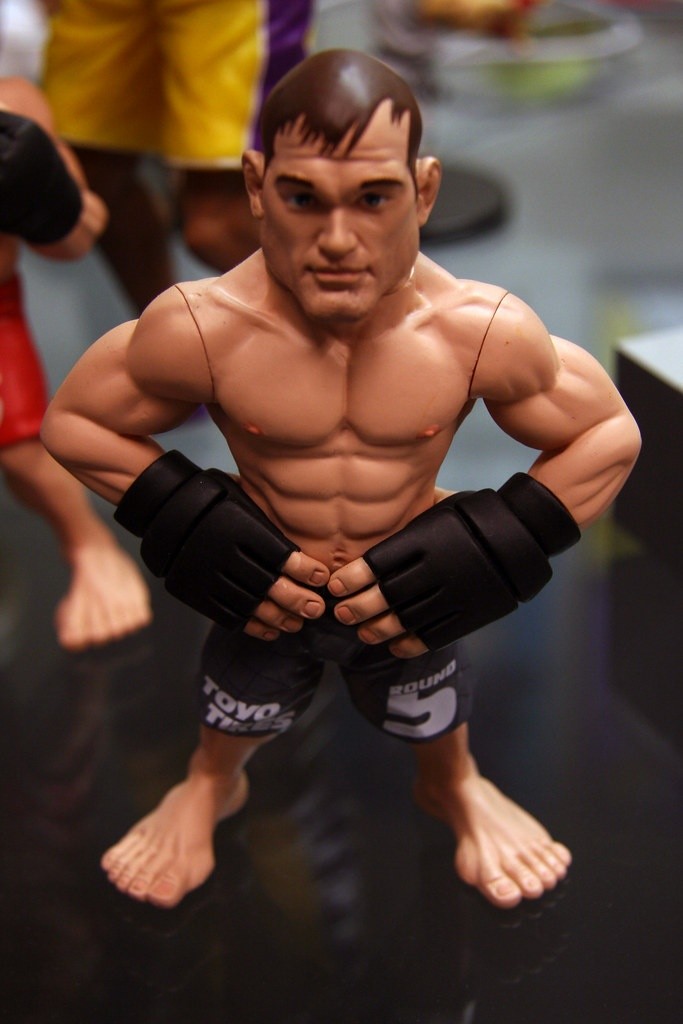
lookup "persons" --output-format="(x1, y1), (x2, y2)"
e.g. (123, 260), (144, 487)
(0, 0), (325, 648)
(41, 49), (642, 907)
